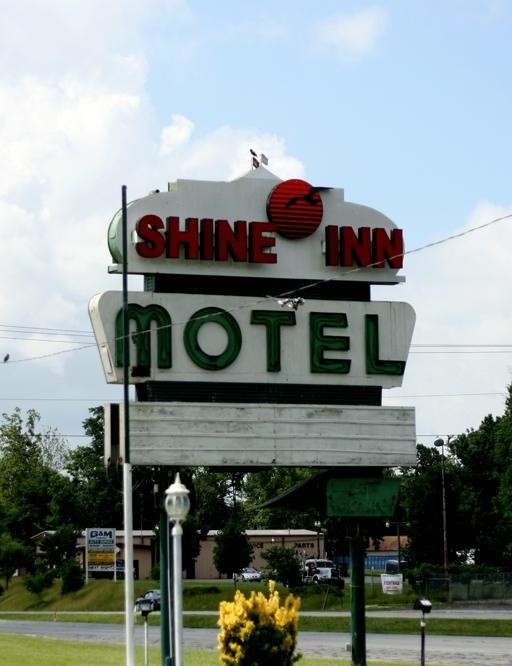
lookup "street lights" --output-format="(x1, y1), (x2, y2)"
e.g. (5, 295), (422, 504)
(385, 519), (412, 574)
(164, 471), (191, 664)
(434, 439), (449, 587)
(313, 521), (321, 557)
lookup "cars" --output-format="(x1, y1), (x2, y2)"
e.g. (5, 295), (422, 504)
(232, 567), (262, 583)
(134, 589), (161, 611)
(299, 558), (343, 583)
